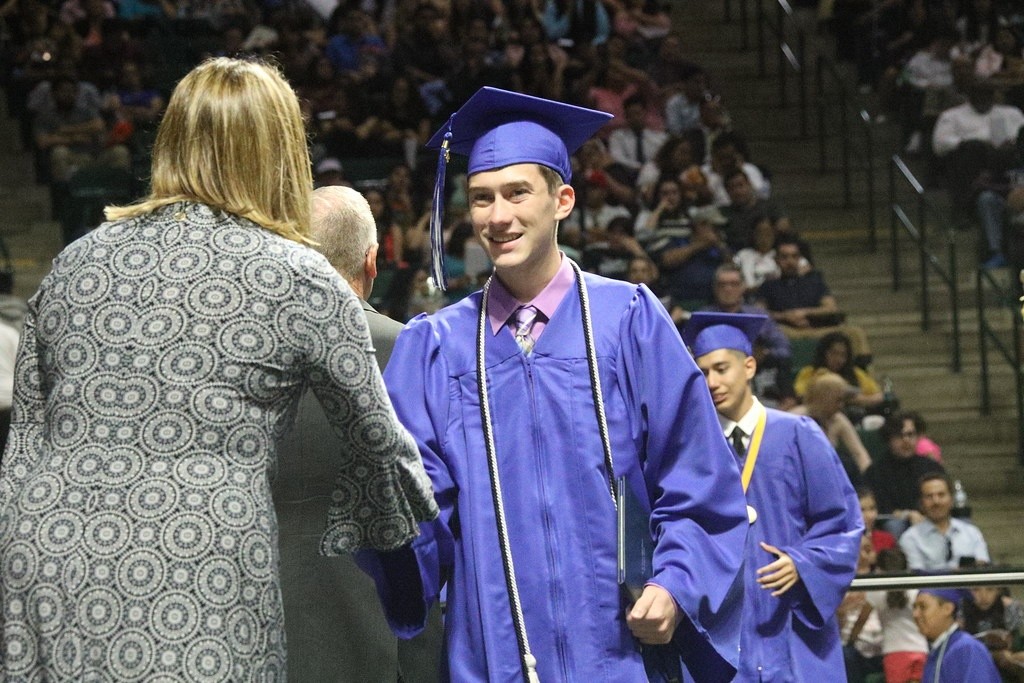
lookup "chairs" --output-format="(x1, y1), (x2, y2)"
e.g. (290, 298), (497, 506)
(0, 0), (1023, 479)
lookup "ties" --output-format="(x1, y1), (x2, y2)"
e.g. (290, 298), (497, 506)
(732, 427), (746, 455)
(513, 307), (537, 354)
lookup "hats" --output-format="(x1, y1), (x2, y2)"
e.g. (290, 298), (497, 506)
(318, 160), (342, 173)
(679, 310), (768, 359)
(912, 569), (974, 607)
(425, 88), (614, 290)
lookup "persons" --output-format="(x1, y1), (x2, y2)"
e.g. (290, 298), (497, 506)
(685, 313), (865, 683)
(0, 0), (1024, 683)
(353, 88), (749, 683)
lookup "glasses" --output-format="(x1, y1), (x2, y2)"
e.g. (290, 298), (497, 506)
(719, 281), (741, 288)
